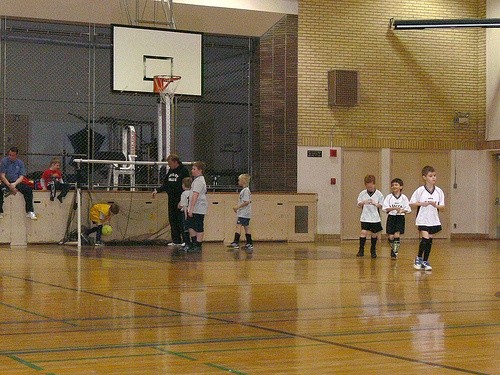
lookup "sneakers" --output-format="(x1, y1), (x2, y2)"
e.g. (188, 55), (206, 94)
(95, 240), (106, 246)
(241, 243), (254, 249)
(25, 212), (37, 221)
(81, 232), (90, 242)
(421, 260), (432, 271)
(390, 250), (398, 260)
(414, 256), (422, 270)
(0, 212), (4, 218)
(226, 242), (239, 249)
(188, 244), (202, 253)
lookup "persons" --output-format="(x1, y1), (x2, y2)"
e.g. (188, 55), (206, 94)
(226, 174), (253, 249)
(40, 159), (70, 203)
(382, 178), (411, 260)
(409, 166), (445, 271)
(81, 203), (119, 246)
(0, 147), (38, 219)
(151, 154), (190, 246)
(355, 175), (385, 258)
(178, 177), (193, 251)
(186, 161), (208, 252)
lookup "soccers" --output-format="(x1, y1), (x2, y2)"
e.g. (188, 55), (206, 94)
(102, 225), (111, 236)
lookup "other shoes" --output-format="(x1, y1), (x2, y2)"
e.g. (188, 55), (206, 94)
(356, 249), (364, 256)
(180, 245), (190, 252)
(50, 197), (55, 201)
(371, 248), (377, 258)
(167, 242), (181, 245)
(179, 242), (186, 246)
(58, 195), (63, 203)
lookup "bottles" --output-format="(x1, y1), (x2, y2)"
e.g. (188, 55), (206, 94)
(34, 178), (37, 190)
